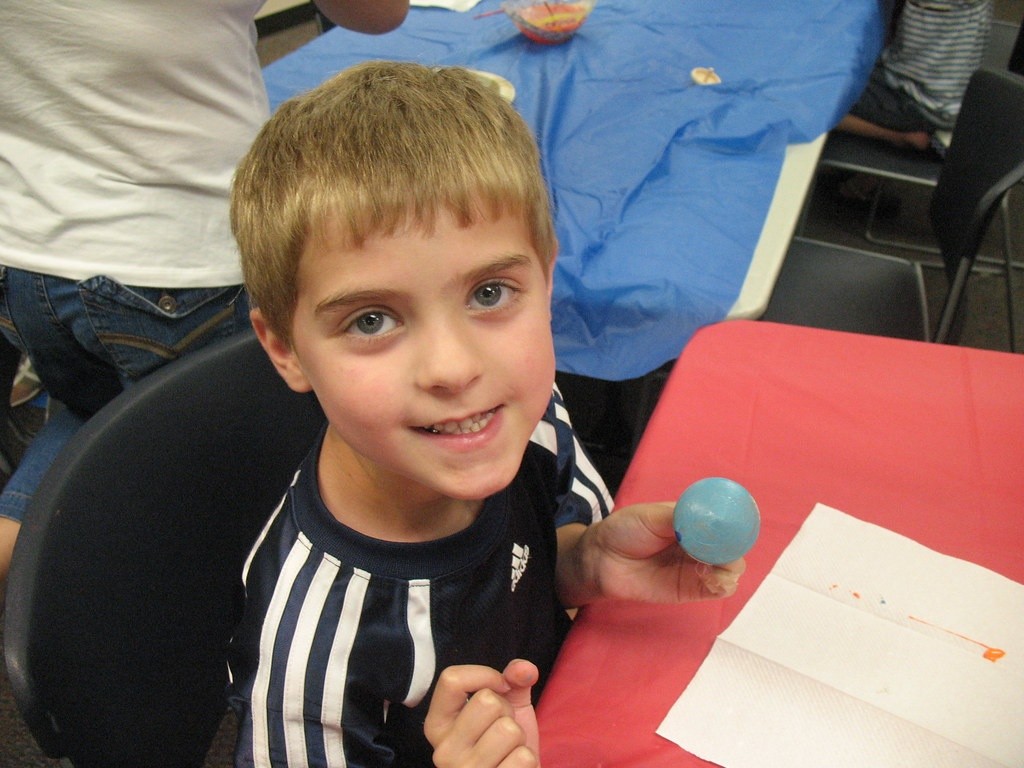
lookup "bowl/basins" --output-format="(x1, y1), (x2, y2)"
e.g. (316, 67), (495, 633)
(499, 0), (596, 43)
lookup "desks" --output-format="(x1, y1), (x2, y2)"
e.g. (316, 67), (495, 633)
(532, 320), (1024, 768)
(261, 1), (883, 453)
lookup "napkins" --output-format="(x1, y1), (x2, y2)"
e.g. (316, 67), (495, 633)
(654, 501), (1024, 768)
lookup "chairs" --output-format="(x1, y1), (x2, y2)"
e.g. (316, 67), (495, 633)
(757, 64), (1024, 345)
(1, 329), (327, 768)
(827, 21), (1024, 271)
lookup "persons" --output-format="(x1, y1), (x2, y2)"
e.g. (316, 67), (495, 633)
(0, 1), (416, 584)
(231, 59), (745, 767)
(832, 1), (995, 151)
